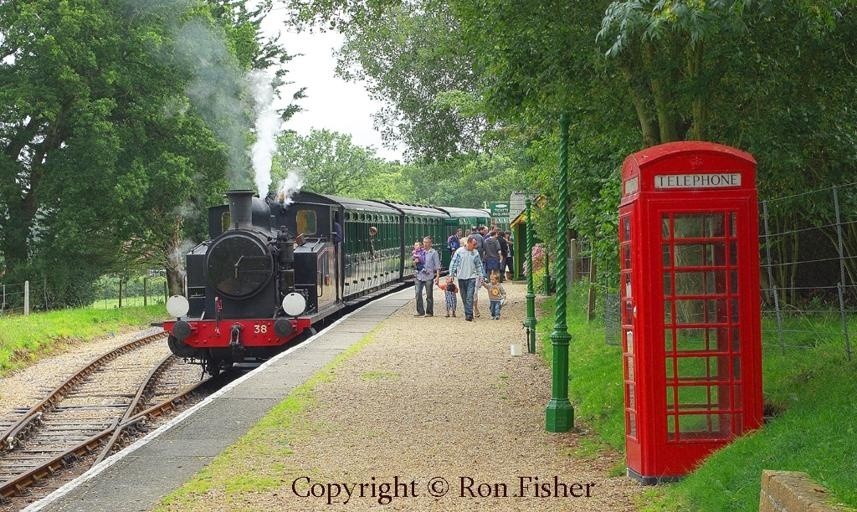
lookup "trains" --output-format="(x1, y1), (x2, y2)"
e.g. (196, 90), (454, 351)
(149, 189), (510, 380)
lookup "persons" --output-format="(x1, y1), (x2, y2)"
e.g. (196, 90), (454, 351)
(412, 242), (430, 276)
(334, 221), (344, 264)
(434, 276), (458, 318)
(448, 236), (485, 322)
(448, 224), (515, 283)
(473, 277), (484, 318)
(413, 236), (442, 317)
(482, 274), (507, 320)
(368, 225), (377, 236)
